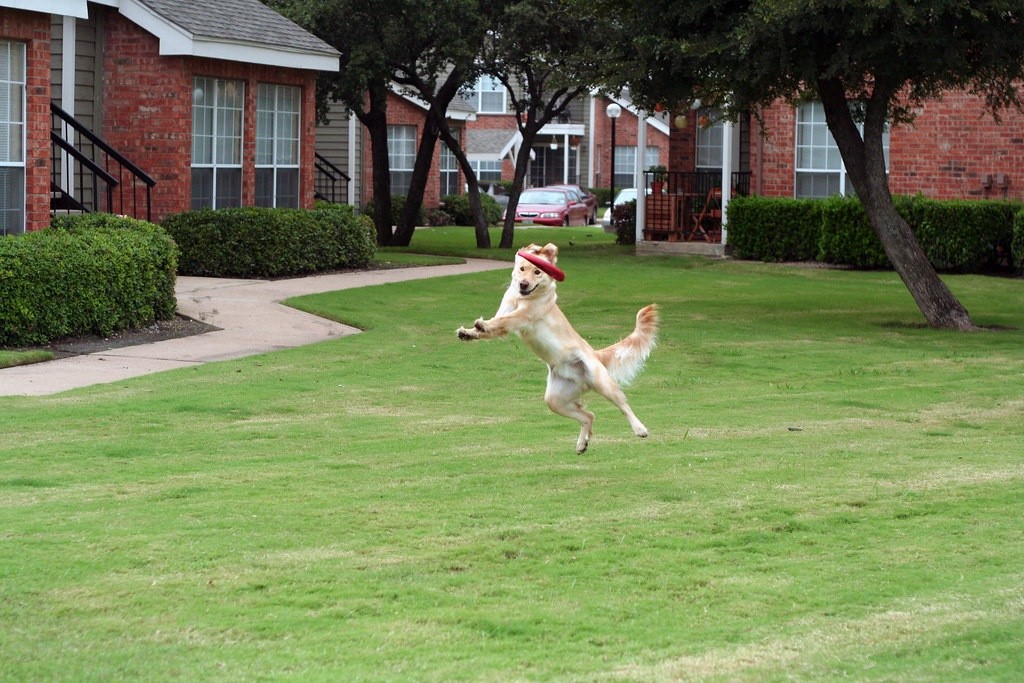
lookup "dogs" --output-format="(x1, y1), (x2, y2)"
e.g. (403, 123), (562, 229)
(456, 243), (656, 455)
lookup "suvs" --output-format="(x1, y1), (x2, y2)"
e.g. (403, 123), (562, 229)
(477, 182), (510, 215)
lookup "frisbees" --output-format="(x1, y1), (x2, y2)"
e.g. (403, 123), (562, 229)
(517, 251), (566, 282)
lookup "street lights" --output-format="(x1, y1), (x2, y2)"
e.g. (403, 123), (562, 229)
(606, 103), (622, 227)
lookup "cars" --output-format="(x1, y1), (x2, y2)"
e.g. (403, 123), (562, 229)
(602, 188), (667, 229)
(547, 185), (598, 225)
(502, 188), (590, 229)
